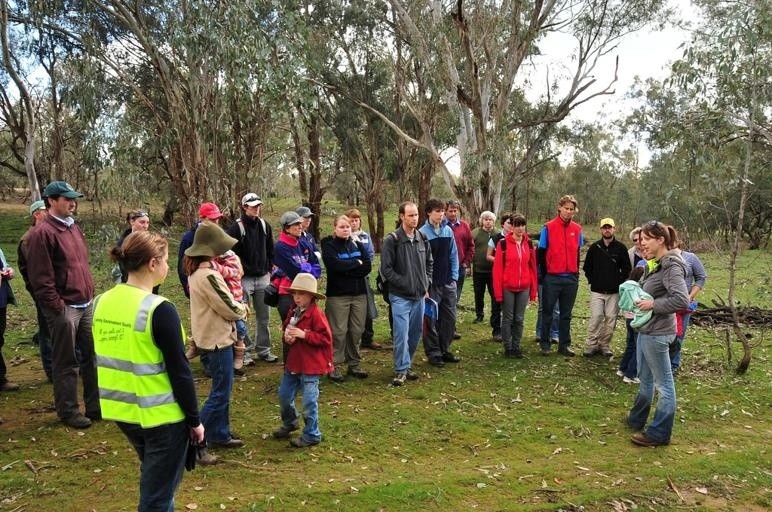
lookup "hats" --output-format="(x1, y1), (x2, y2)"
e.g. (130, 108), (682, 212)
(600, 217), (615, 227)
(30, 181), (314, 259)
(283, 273), (326, 301)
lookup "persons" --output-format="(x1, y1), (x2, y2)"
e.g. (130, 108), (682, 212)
(178, 193), (382, 380)
(273, 273), (336, 448)
(583, 218), (705, 446)
(184, 218), (249, 466)
(2, 182), (150, 427)
(379, 196), (583, 387)
(77, 233), (206, 511)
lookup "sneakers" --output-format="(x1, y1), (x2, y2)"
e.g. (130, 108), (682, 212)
(61, 413), (92, 428)
(1, 383), (18, 389)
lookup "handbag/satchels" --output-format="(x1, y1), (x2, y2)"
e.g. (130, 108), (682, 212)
(263, 285), (278, 307)
